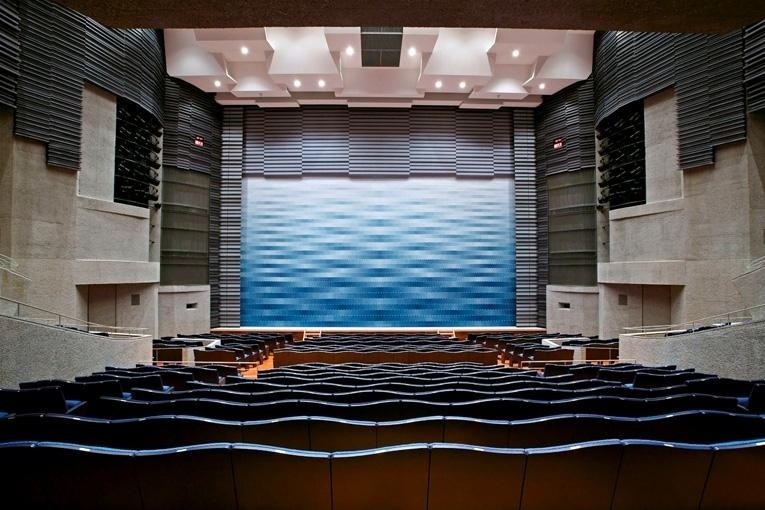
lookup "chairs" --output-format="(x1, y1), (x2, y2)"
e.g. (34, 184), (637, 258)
(1, 333), (765, 510)
